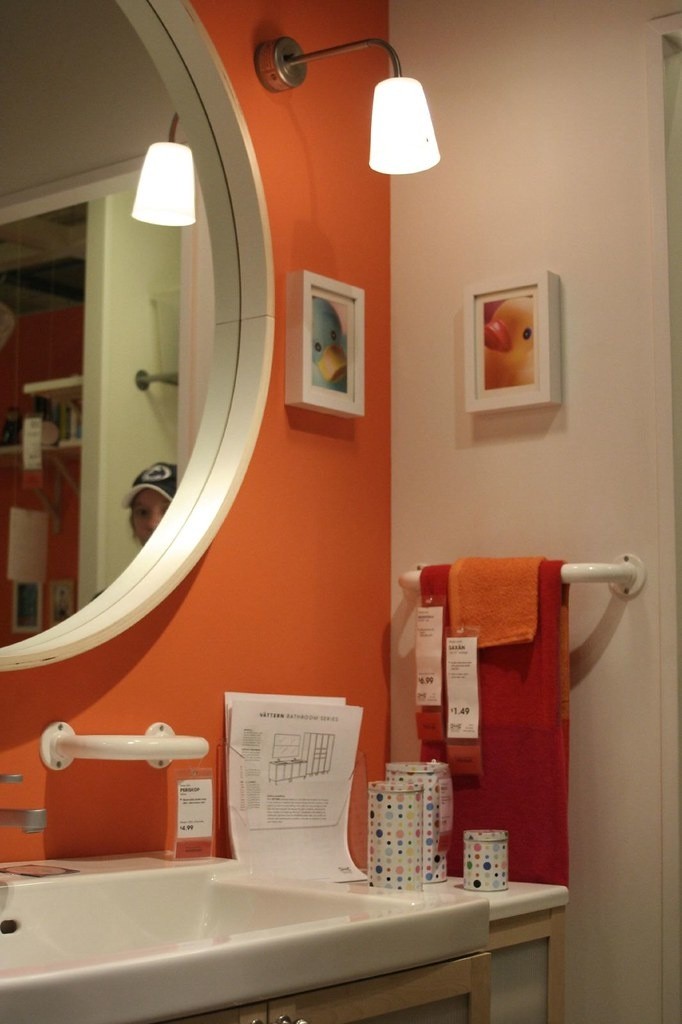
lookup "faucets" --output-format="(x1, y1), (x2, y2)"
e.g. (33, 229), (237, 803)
(0, 774), (46, 837)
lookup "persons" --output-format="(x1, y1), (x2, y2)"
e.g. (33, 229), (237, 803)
(122, 462), (177, 547)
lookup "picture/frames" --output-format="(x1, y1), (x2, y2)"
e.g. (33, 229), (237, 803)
(462, 270), (564, 412)
(285, 271), (365, 416)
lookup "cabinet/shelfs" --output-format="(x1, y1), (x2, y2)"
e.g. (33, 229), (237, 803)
(168, 909), (568, 1023)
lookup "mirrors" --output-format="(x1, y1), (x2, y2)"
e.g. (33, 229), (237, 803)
(0, 0), (277, 677)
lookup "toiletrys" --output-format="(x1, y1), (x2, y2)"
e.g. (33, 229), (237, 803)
(168, 758), (216, 864)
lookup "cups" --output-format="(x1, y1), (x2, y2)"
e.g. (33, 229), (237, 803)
(365, 780), (424, 894)
(462, 828), (509, 892)
(385, 760), (451, 884)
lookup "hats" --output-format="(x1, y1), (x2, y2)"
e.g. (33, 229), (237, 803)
(123, 461), (177, 508)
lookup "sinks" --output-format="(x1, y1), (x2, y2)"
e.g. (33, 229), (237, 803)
(0, 849), (489, 1024)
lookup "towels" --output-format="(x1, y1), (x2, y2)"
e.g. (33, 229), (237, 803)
(415, 557), (569, 885)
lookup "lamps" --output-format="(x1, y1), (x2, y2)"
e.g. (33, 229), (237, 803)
(251, 35), (440, 175)
(132, 113), (197, 227)
